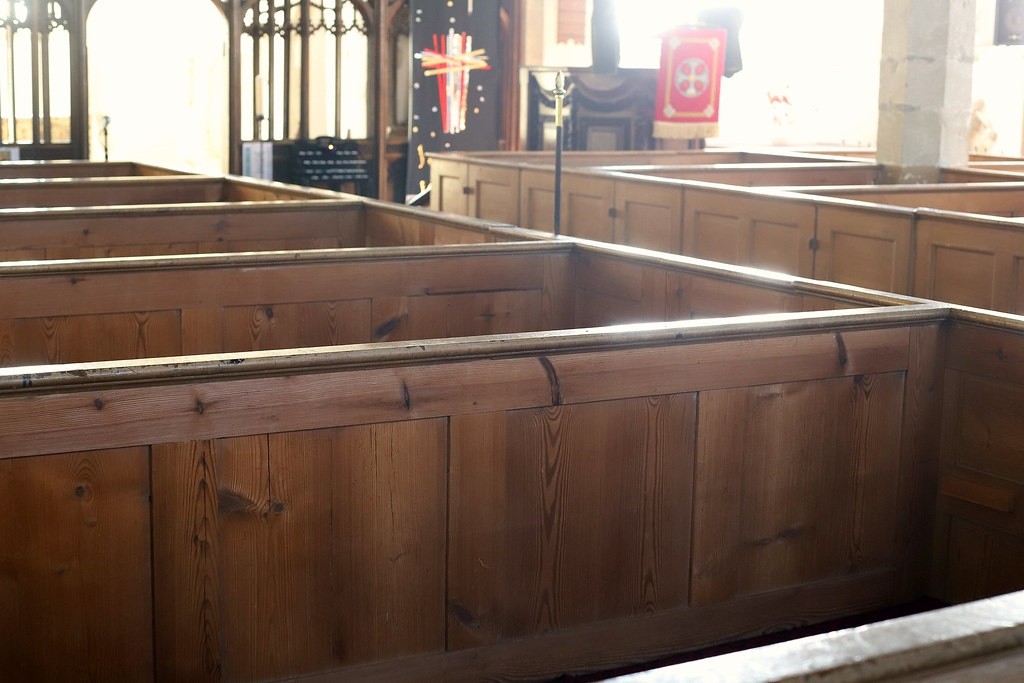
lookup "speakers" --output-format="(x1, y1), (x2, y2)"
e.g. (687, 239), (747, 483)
(994, 0), (1024, 46)
